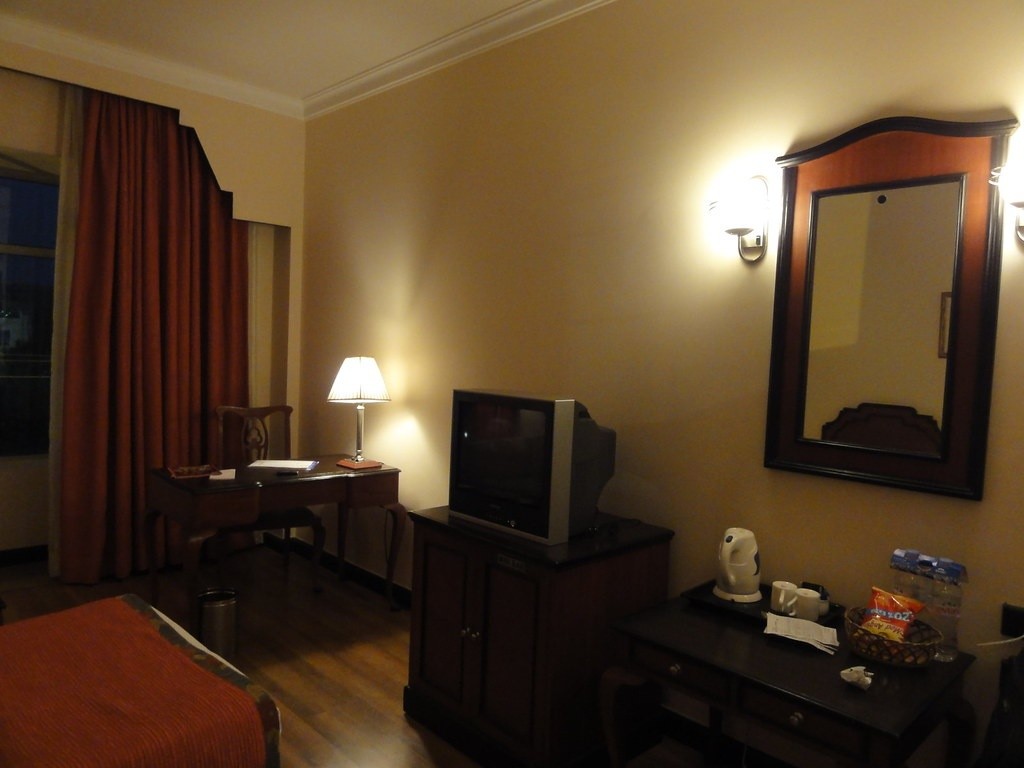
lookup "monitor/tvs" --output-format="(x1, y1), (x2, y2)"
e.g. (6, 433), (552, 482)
(448, 388), (616, 545)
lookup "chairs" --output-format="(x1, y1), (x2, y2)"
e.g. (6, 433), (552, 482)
(214, 403), (326, 593)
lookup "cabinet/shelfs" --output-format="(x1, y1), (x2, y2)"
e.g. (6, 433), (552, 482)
(401, 501), (677, 768)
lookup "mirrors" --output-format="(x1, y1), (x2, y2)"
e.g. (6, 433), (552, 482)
(764, 114), (1018, 502)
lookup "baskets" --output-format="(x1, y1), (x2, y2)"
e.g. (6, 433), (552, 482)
(845, 606), (942, 667)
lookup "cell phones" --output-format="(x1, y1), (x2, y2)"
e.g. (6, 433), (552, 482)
(275, 471), (299, 476)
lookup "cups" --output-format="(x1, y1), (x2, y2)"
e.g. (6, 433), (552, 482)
(770, 580), (798, 612)
(787, 587), (821, 621)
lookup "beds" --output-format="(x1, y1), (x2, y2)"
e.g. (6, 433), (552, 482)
(0, 592), (284, 768)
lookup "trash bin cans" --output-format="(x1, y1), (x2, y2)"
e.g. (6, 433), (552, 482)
(197, 585), (240, 660)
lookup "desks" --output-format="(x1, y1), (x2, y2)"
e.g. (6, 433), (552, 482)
(610, 580), (978, 768)
(143, 454), (402, 634)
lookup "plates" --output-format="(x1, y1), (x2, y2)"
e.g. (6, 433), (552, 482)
(167, 463), (222, 479)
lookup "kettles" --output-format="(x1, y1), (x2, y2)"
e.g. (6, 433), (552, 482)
(712, 527), (763, 603)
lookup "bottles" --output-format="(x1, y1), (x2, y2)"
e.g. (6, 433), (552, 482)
(931, 557), (963, 663)
(893, 549), (922, 600)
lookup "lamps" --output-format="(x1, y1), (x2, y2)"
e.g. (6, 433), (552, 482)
(716, 163), (772, 264)
(326, 357), (392, 473)
(997, 133), (1024, 241)
